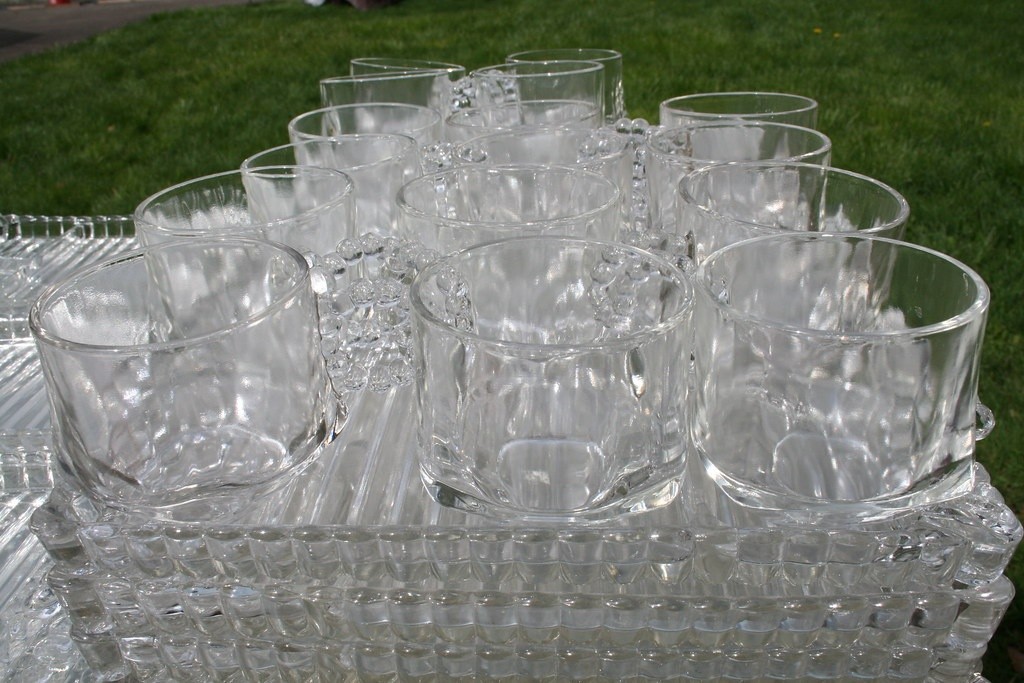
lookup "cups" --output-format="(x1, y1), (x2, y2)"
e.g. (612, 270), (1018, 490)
(474, 61), (607, 128)
(645, 120), (833, 241)
(133, 165), (356, 297)
(444, 99), (601, 143)
(450, 128), (632, 208)
(408, 236), (695, 527)
(659, 92), (818, 160)
(396, 164), (621, 260)
(674, 160), (911, 318)
(29, 236), (340, 521)
(287, 102), (442, 171)
(695, 232), (991, 517)
(242, 134), (425, 235)
(350, 57), (467, 102)
(506, 48), (626, 126)
(319, 71), (453, 137)
